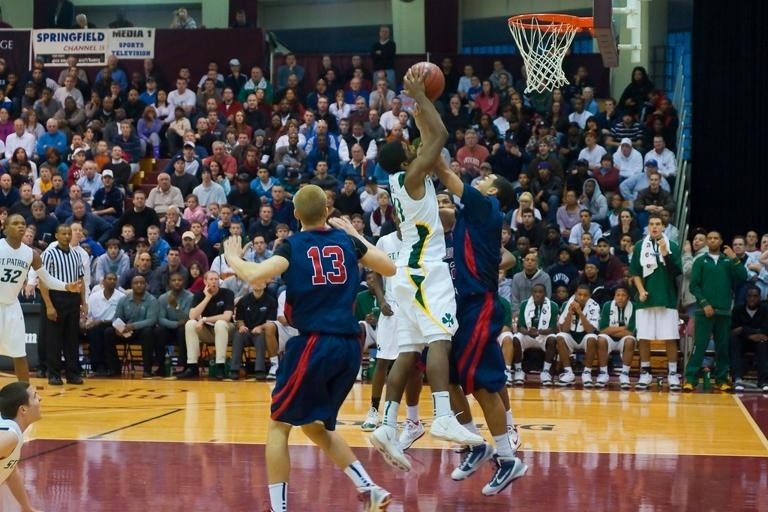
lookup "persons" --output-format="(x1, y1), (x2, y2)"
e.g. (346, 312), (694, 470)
(1, 381), (43, 510)
(361, 67), (530, 495)
(1, 9), (767, 391)
(222, 181), (396, 511)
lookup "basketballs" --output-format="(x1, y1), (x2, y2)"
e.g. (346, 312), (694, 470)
(405, 62), (445, 102)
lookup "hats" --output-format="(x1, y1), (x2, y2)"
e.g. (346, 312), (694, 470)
(181, 230), (195, 240)
(620, 137), (632, 147)
(230, 58), (240, 66)
(136, 237), (150, 247)
(183, 140), (195, 148)
(105, 238), (120, 246)
(101, 169), (113, 178)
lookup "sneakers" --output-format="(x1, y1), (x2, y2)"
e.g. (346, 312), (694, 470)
(174, 366), (278, 380)
(539, 369), (552, 386)
(504, 369), (514, 386)
(358, 407), (529, 511)
(48, 373), (63, 385)
(618, 375), (632, 389)
(594, 374), (609, 388)
(581, 373), (593, 388)
(555, 373), (577, 386)
(65, 373), (83, 384)
(514, 368), (526, 385)
(633, 374), (652, 390)
(682, 382), (767, 392)
(667, 373), (681, 390)
